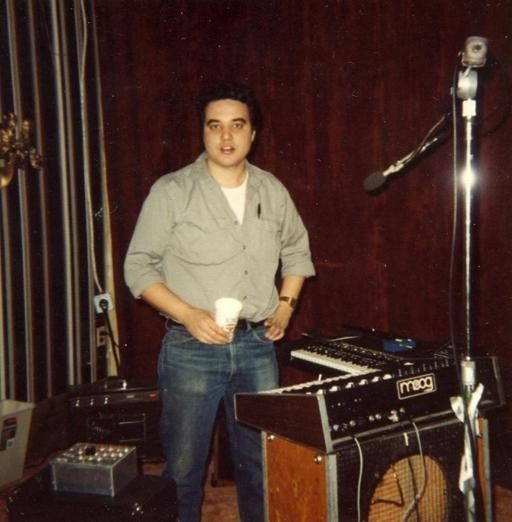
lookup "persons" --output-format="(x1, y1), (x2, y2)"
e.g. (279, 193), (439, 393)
(120, 79), (318, 521)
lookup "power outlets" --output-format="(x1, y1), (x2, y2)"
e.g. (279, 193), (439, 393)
(96, 325), (106, 347)
(92, 291), (115, 314)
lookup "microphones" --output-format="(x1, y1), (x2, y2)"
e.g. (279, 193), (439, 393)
(363, 117), (460, 191)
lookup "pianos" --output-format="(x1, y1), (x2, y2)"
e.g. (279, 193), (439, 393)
(279, 325), (443, 374)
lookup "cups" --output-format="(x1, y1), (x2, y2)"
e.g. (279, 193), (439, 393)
(213, 296), (241, 345)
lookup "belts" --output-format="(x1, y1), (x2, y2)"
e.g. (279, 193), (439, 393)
(168, 315), (268, 330)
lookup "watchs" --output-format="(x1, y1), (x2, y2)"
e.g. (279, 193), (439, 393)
(278, 296), (299, 308)
